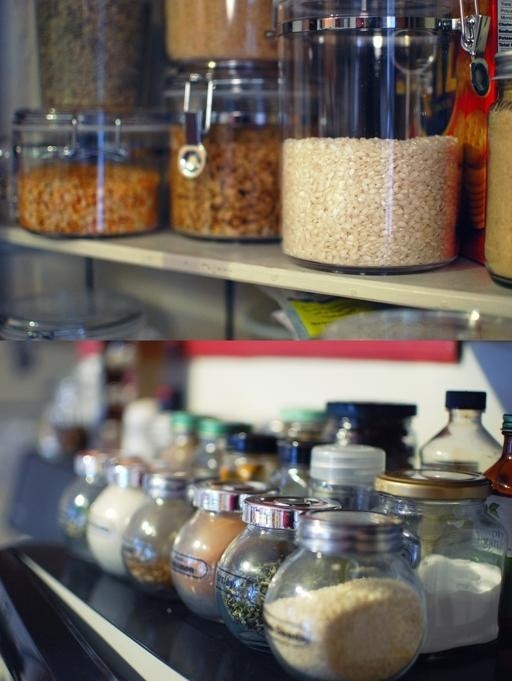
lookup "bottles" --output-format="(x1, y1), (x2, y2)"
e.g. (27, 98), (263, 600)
(269, 0), (493, 278)
(57, 388), (512, 680)
(166, 56), (282, 246)
(11, 103), (169, 242)
(482, 49), (512, 292)
